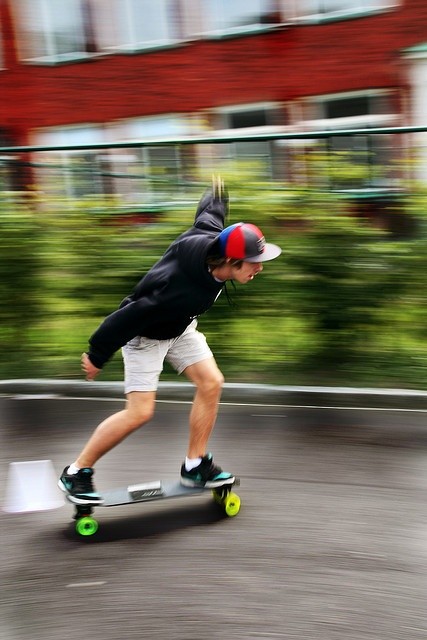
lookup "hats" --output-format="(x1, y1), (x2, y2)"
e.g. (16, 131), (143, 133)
(219, 221), (282, 262)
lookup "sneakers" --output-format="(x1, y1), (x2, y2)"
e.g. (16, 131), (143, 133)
(58, 465), (104, 506)
(180, 452), (236, 490)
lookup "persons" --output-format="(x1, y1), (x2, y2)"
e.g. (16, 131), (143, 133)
(56, 174), (282, 508)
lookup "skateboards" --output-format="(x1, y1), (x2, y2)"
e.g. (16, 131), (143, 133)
(64, 476), (243, 538)
